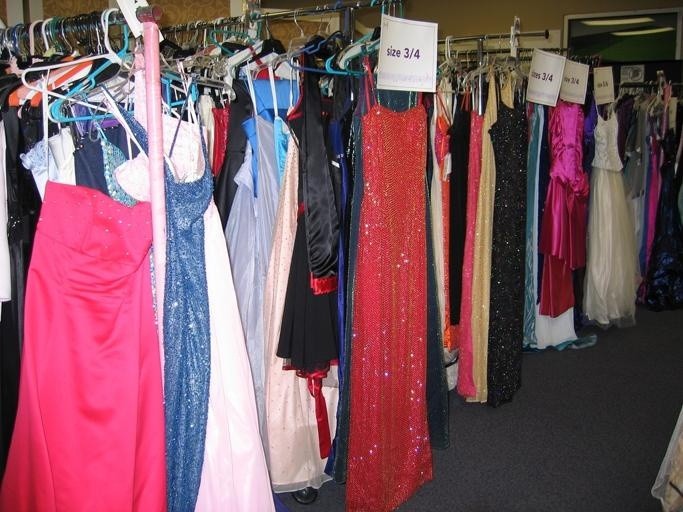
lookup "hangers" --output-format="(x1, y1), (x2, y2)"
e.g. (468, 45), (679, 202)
(200, 0), (406, 117)
(2, 9), (181, 127)
(418, 22), (683, 118)
